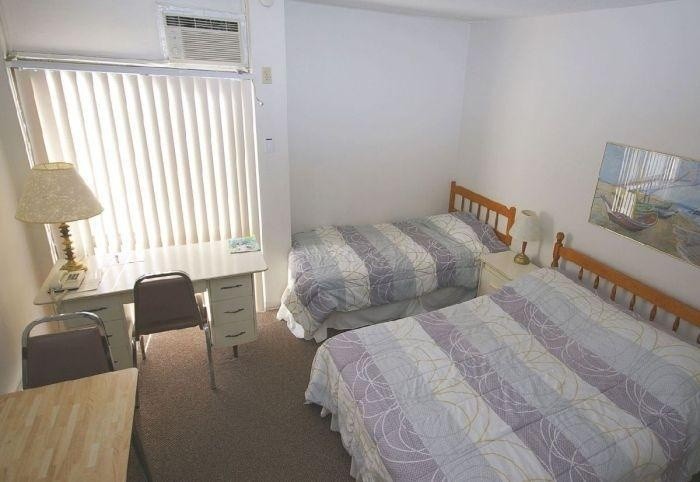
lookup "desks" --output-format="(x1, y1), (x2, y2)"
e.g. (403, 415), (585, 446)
(1, 368), (156, 481)
(33, 233), (270, 374)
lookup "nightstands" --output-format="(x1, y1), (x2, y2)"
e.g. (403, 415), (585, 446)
(477, 250), (541, 299)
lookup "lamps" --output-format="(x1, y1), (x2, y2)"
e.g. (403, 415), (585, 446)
(15, 161), (105, 272)
(508, 208), (543, 265)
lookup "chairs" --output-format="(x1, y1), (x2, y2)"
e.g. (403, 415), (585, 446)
(18, 311), (118, 393)
(126, 268), (222, 395)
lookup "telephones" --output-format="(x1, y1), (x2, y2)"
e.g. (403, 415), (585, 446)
(48, 269), (85, 292)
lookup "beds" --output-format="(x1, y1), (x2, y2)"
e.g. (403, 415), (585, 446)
(302, 228), (699, 482)
(276, 179), (517, 342)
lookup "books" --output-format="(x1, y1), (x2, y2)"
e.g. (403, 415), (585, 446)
(227, 236), (261, 253)
